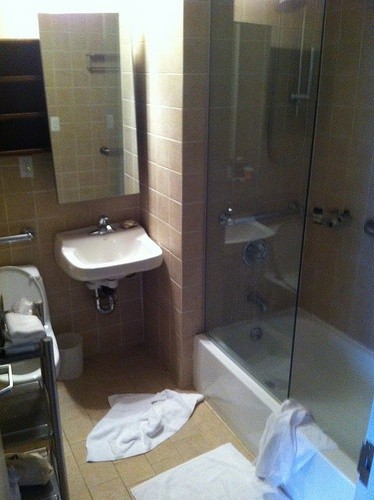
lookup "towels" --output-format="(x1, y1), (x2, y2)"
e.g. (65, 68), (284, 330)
(255, 399), (308, 485)
(85, 388), (205, 463)
(3, 312), (47, 355)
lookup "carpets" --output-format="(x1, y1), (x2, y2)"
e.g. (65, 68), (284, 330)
(131, 442), (291, 500)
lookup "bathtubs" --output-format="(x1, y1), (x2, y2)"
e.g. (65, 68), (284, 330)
(192, 307), (374, 500)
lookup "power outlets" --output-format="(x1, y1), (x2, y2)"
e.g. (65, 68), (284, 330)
(20, 155), (34, 178)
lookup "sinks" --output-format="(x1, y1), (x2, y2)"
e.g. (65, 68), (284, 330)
(54, 220), (165, 282)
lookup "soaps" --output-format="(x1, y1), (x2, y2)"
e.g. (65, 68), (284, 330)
(124, 219), (135, 226)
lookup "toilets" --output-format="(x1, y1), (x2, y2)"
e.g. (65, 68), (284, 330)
(0, 264), (61, 391)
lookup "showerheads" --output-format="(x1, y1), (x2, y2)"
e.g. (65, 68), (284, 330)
(279, 0), (306, 10)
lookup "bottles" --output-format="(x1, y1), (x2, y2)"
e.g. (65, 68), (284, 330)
(32, 300), (44, 326)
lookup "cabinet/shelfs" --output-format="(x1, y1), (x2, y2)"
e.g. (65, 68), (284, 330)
(0, 301), (71, 500)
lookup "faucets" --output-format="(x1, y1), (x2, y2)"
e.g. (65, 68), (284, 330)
(97, 216), (113, 233)
(247, 292), (267, 312)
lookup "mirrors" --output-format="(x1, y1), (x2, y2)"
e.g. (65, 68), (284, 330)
(37, 10), (141, 204)
